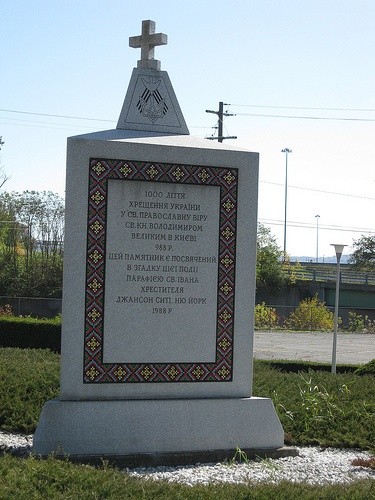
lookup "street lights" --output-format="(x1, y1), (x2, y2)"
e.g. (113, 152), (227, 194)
(330, 243), (349, 375)
(315, 215), (320, 263)
(281, 147), (292, 261)
